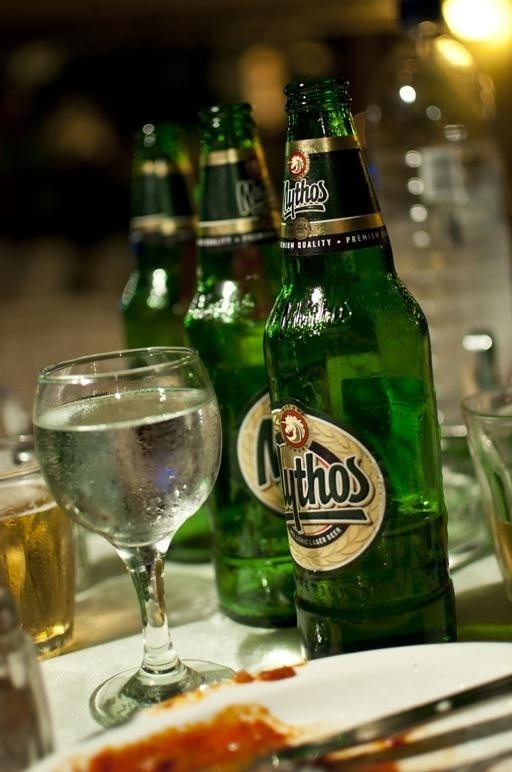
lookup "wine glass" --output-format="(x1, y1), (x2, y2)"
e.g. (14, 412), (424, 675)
(28, 345), (250, 731)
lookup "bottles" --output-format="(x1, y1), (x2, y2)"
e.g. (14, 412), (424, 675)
(314, 1), (510, 443)
(179, 101), (291, 628)
(120, 94), (215, 566)
(260, 71), (457, 662)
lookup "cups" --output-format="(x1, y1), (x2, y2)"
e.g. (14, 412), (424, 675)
(460, 379), (512, 617)
(2, 426), (87, 668)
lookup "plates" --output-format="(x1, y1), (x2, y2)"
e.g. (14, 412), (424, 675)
(26, 642), (511, 772)
